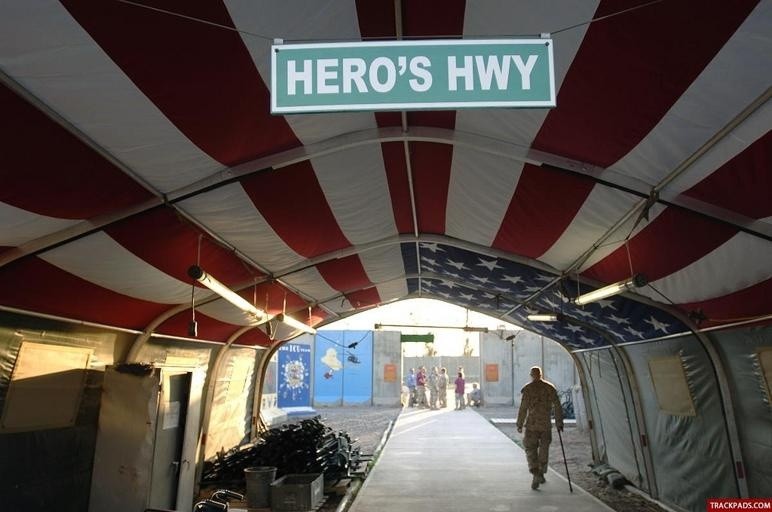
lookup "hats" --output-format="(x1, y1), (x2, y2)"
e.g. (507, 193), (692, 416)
(530, 366), (541, 376)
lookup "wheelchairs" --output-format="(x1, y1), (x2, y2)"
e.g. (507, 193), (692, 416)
(142, 488), (247, 512)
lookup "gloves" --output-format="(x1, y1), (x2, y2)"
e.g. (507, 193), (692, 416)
(518, 427), (522, 432)
(558, 426), (563, 432)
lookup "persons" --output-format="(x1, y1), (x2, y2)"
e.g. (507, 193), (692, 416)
(458, 366), (465, 380)
(516, 367), (565, 489)
(455, 372), (464, 410)
(406, 366), (449, 410)
(466, 382), (480, 406)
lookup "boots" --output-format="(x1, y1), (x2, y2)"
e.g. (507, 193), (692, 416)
(532, 470), (545, 489)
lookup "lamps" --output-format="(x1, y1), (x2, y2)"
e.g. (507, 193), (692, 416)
(524, 239), (645, 324)
(187, 236), (319, 337)
